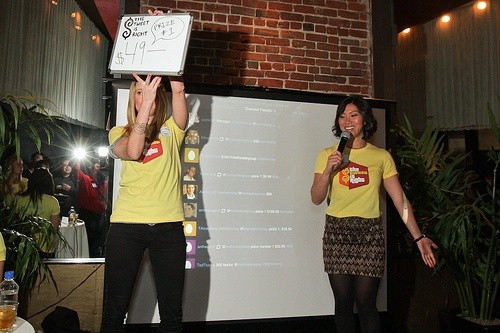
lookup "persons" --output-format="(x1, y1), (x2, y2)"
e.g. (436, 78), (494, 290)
(100, 10), (188, 333)
(52, 156), (75, 220)
(22, 153), (50, 179)
(184, 184), (197, 200)
(11, 167), (60, 258)
(311, 94), (438, 333)
(38, 306), (80, 333)
(0, 231), (6, 286)
(183, 166), (197, 182)
(2, 157), (28, 210)
(71, 158), (108, 258)
(186, 204), (195, 218)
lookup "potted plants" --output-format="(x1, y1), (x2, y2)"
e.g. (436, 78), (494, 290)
(389, 103), (500, 333)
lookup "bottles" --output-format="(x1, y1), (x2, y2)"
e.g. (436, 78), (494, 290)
(0, 271), (19, 327)
(68, 206), (76, 227)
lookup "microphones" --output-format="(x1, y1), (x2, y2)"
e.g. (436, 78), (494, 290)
(336, 131), (352, 157)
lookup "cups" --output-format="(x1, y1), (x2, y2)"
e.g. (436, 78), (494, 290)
(0, 300), (19, 333)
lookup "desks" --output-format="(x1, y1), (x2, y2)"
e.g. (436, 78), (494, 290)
(56, 217), (90, 257)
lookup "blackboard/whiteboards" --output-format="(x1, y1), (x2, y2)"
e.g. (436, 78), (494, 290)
(108, 12), (194, 79)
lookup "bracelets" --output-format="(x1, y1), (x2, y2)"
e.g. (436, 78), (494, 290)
(415, 234), (425, 242)
